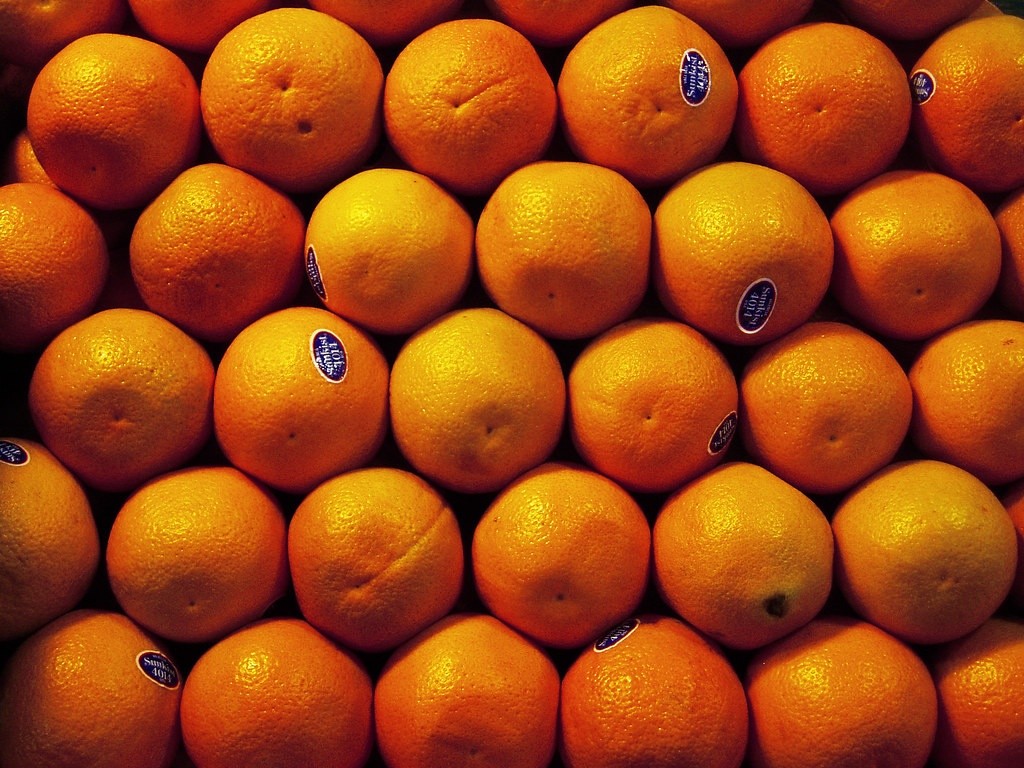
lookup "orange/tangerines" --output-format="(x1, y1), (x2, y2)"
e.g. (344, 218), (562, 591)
(0, 0), (1024, 768)
